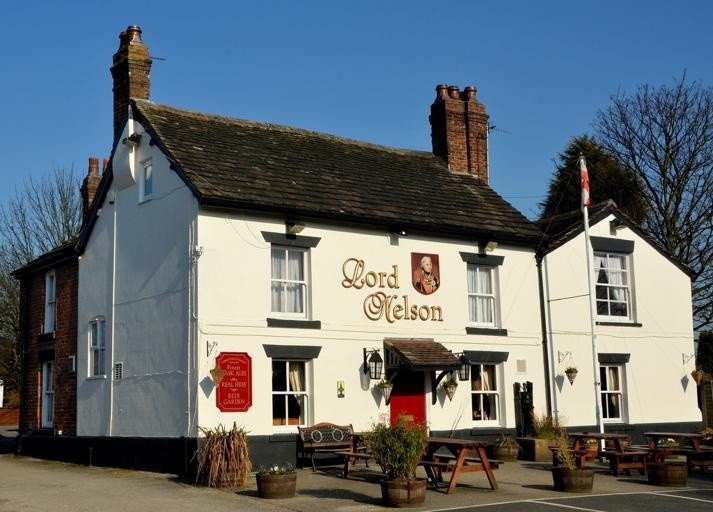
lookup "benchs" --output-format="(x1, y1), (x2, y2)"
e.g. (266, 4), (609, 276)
(335, 450), (505, 483)
(297, 422), (369, 472)
(636, 444), (712, 472)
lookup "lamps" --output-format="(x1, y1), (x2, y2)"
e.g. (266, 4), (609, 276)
(448, 349), (471, 381)
(124, 132), (142, 148)
(362, 347), (383, 380)
(478, 239), (498, 252)
(609, 217), (627, 231)
(284, 220), (306, 234)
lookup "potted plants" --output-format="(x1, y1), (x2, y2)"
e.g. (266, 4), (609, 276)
(441, 378), (458, 402)
(493, 410), (594, 493)
(690, 365), (705, 386)
(564, 367), (577, 386)
(644, 459), (689, 486)
(357, 412), (430, 507)
(188, 419), (297, 499)
(375, 374), (393, 405)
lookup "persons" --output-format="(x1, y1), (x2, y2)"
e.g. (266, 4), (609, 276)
(413, 256), (438, 294)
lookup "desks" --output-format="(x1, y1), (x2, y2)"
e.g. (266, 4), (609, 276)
(349, 432), (498, 495)
(567, 432), (707, 477)
